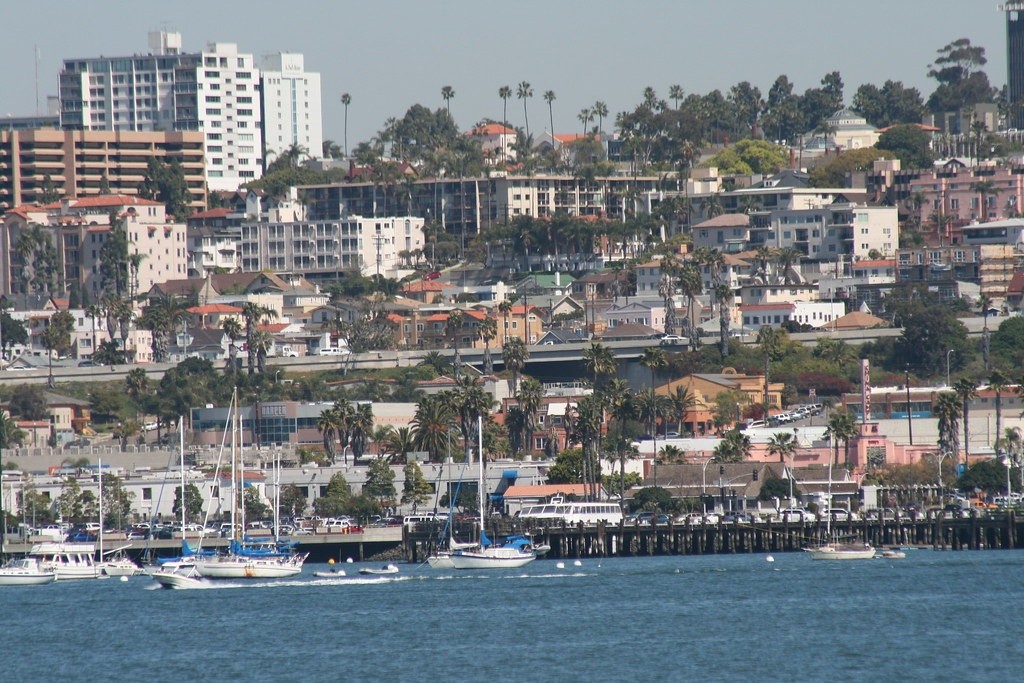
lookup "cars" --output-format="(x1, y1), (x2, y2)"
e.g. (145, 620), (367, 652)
(819, 507), (858, 522)
(293, 527), (317, 536)
(633, 433), (653, 442)
(626, 509), (763, 527)
(942, 490), (1024, 517)
(313, 507), (402, 534)
(742, 401), (825, 432)
(78, 361), (103, 367)
(656, 431), (682, 441)
(770, 506), (817, 523)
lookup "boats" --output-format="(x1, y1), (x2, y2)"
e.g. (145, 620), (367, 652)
(873, 550), (907, 560)
(313, 568), (347, 578)
(357, 563), (400, 575)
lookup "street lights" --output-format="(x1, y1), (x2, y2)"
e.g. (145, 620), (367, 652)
(702, 456), (716, 524)
(939, 452), (953, 513)
(947, 349), (955, 386)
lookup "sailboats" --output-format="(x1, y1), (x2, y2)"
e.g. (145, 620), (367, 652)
(0, 385), (310, 591)
(800, 430), (877, 561)
(425, 406), (550, 569)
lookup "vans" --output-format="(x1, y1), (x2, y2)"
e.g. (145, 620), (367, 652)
(404, 515), (438, 527)
(319, 347), (353, 356)
